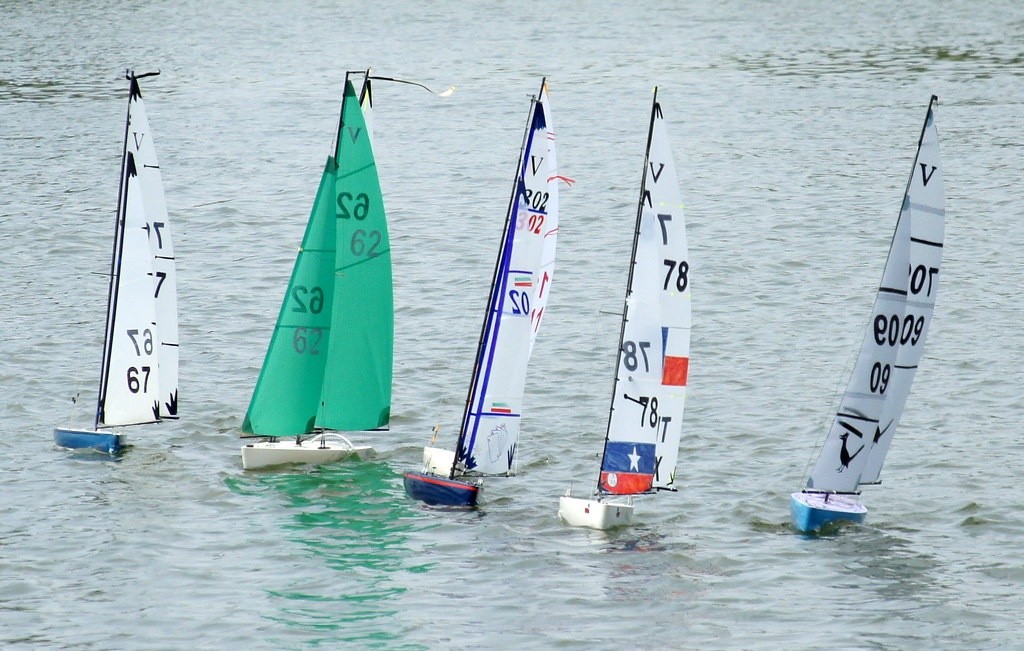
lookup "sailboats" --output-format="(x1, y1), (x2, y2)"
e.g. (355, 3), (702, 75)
(50, 70), (181, 464)
(402, 75), (576, 513)
(789, 90), (949, 539)
(237, 68), (461, 479)
(556, 83), (693, 532)
